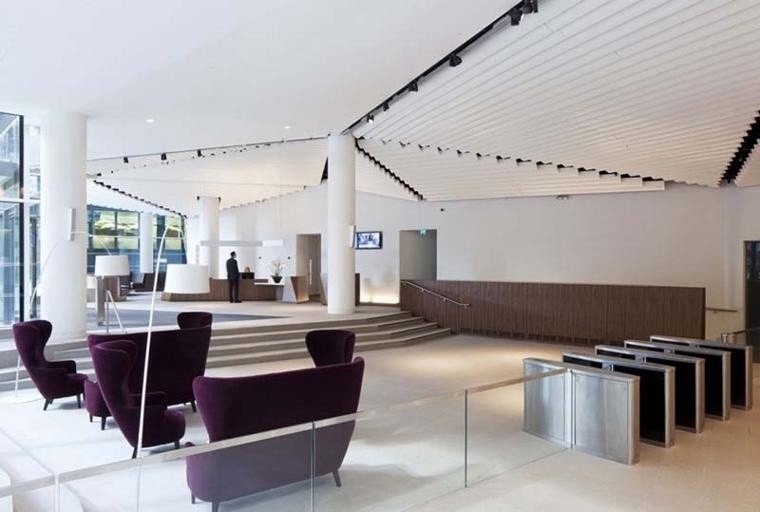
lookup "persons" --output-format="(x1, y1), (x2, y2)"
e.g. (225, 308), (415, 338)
(226, 251), (243, 304)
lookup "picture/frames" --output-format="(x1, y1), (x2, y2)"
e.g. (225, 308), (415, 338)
(357, 231), (382, 250)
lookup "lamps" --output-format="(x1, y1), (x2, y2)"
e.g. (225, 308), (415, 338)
(0, 230), (130, 405)
(135, 221), (211, 458)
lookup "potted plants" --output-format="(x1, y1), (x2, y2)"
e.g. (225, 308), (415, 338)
(270, 258), (286, 283)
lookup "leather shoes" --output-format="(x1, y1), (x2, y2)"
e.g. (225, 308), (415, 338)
(230, 300), (242, 303)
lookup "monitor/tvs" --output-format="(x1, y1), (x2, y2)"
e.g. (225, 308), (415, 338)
(242, 272), (254, 279)
(355, 231), (381, 249)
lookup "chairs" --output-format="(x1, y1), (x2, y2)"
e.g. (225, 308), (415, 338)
(12, 320), (89, 410)
(305, 329), (355, 367)
(177, 311), (213, 329)
(92, 340), (186, 459)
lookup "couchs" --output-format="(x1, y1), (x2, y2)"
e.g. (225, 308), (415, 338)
(186, 356), (365, 508)
(84, 325), (212, 430)
